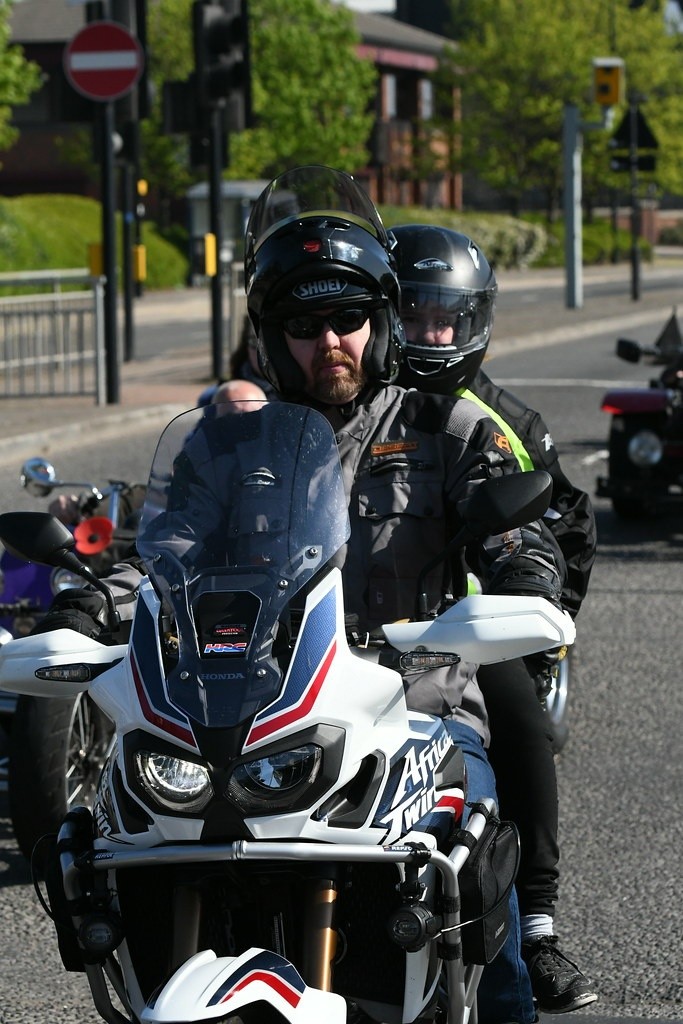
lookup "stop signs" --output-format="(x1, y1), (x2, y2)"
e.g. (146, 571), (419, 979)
(66, 21), (143, 102)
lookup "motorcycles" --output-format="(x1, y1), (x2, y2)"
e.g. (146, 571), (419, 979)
(0, 454), (179, 874)
(594, 307), (683, 537)
(0, 399), (579, 1024)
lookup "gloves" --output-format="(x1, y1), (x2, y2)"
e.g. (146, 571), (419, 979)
(496, 590), (571, 677)
(27, 588), (104, 639)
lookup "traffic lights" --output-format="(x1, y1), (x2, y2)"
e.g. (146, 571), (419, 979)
(192, 1), (253, 109)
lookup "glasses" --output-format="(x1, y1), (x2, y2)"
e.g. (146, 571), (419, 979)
(279, 307), (373, 341)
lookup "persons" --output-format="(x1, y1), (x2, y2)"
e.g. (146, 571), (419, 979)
(381, 224), (598, 621)
(48, 380), (270, 532)
(28, 164), (600, 1016)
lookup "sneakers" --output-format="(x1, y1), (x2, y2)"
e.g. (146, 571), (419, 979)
(521, 934), (599, 1013)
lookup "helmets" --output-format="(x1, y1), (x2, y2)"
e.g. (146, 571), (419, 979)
(244, 214), (402, 403)
(386, 223), (498, 396)
